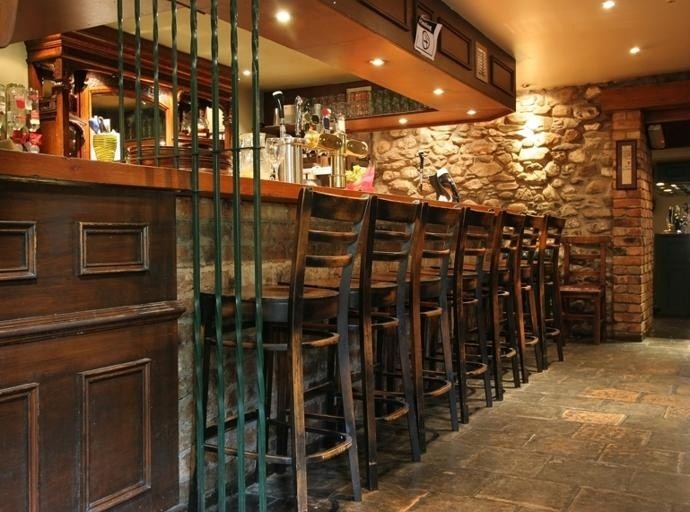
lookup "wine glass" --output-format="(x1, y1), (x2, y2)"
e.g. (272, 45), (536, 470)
(268, 139), (285, 181)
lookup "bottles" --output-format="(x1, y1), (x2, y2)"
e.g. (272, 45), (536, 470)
(0, 81), (44, 142)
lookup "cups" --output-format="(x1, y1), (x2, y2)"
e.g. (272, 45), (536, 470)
(93, 134), (117, 162)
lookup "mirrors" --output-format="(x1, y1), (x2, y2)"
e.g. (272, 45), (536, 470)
(654, 177), (690, 237)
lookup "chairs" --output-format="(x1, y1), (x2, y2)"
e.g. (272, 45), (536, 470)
(199, 186), (371, 510)
(453, 211), (526, 400)
(560, 235), (612, 346)
(377, 204), (466, 453)
(275, 195), (424, 490)
(523, 214), (567, 372)
(430, 207), (500, 423)
(499, 212), (549, 384)
